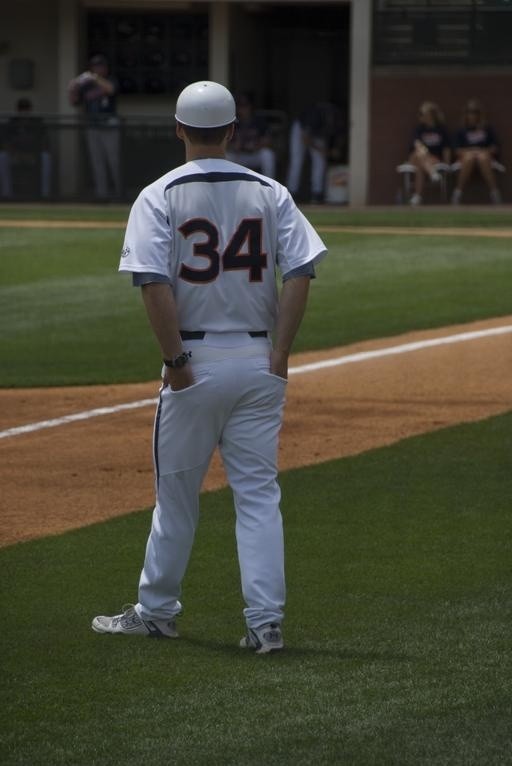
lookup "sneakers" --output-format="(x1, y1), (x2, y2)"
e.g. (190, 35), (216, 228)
(240, 623), (285, 654)
(92, 603), (181, 639)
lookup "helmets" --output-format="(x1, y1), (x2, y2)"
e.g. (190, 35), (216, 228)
(174, 81), (236, 128)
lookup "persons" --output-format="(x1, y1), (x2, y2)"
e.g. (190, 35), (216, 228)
(409, 101), (451, 205)
(451, 100), (504, 205)
(288, 103), (348, 204)
(0, 98), (52, 201)
(91, 80), (328, 656)
(226, 95), (275, 178)
(68, 55), (122, 204)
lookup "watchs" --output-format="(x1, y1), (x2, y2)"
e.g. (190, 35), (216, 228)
(164, 354), (187, 371)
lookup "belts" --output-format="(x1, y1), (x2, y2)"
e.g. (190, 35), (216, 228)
(180, 331), (267, 340)
